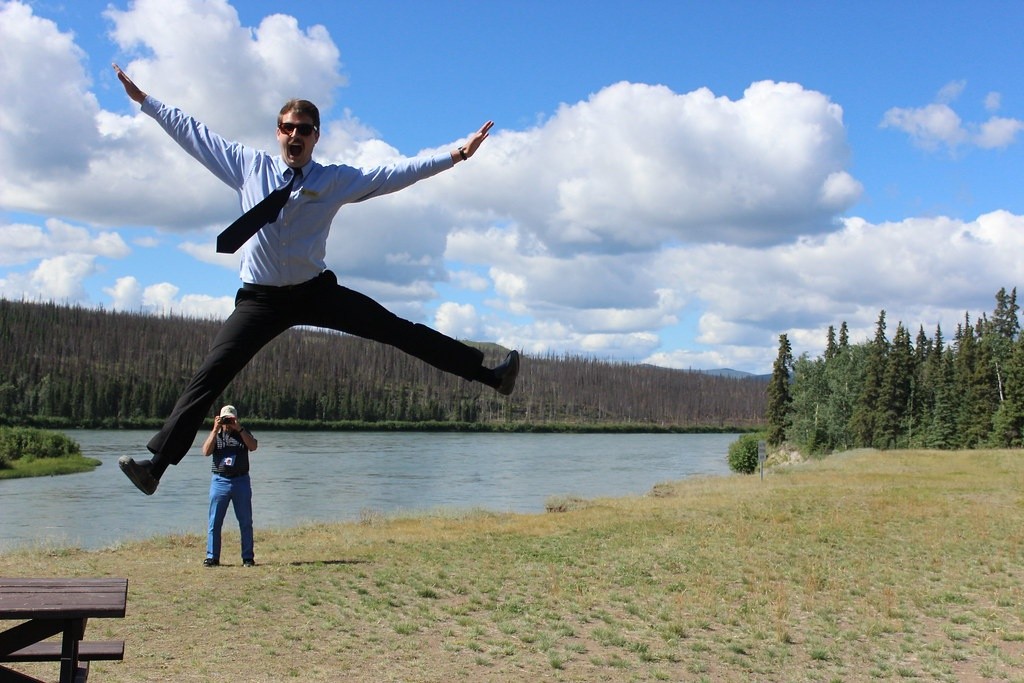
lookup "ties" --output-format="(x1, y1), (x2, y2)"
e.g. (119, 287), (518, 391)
(217, 168), (299, 255)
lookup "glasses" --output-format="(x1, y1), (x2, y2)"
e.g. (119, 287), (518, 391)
(278, 122), (319, 137)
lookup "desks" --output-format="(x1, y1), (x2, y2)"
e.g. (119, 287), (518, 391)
(0, 576), (128, 683)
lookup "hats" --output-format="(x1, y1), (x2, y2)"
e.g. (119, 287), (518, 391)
(220, 405), (237, 421)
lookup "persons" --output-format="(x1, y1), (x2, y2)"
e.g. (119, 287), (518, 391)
(201, 404), (257, 567)
(111, 60), (521, 498)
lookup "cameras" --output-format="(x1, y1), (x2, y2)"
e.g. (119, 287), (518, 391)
(221, 416), (232, 424)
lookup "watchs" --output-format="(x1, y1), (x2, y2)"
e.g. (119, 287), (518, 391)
(238, 428), (244, 433)
(457, 145), (467, 160)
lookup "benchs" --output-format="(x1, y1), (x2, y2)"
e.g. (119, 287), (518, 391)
(0, 640), (125, 663)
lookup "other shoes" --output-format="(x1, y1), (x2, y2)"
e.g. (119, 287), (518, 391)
(243, 558), (254, 568)
(492, 350), (520, 396)
(119, 455), (159, 495)
(204, 558), (219, 567)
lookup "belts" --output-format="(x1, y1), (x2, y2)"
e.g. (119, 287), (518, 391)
(214, 470), (248, 479)
